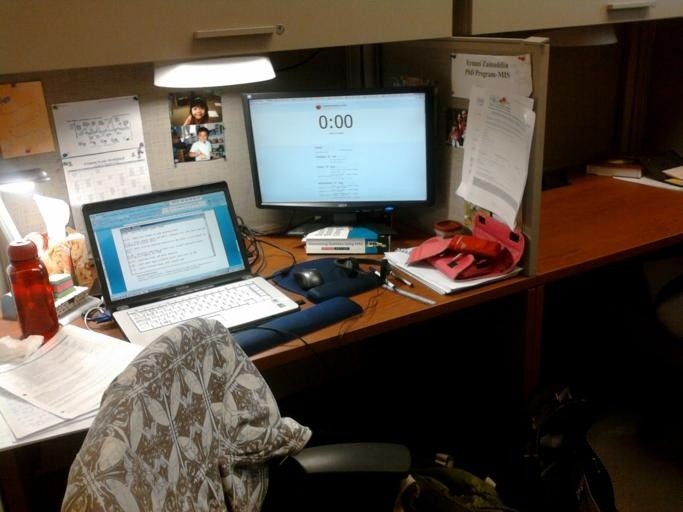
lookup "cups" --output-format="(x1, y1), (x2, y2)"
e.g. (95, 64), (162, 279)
(433, 219), (464, 257)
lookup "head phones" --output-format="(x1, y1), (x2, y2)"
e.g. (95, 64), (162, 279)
(333, 256), (390, 281)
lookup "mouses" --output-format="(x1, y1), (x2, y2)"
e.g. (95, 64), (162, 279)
(293, 268), (324, 290)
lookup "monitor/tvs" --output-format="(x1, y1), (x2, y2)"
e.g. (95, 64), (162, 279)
(239, 86), (434, 238)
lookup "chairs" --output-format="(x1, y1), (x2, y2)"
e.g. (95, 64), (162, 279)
(57, 310), (423, 512)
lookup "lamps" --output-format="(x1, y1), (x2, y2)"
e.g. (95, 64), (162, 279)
(0, 167), (104, 327)
(149, 55), (279, 93)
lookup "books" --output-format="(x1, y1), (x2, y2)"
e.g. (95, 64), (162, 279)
(304, 225), (391, 255)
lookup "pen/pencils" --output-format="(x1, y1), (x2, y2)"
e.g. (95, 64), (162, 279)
(370, 266), (396, 289)
(382, 284), (436, 305)
(390, 270), (412, 287)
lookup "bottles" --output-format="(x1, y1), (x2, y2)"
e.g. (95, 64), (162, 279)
(3, 239), (59, 340)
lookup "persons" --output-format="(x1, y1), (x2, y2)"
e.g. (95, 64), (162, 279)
(182, 99), (209, 124)
(188, 127), (213, 160)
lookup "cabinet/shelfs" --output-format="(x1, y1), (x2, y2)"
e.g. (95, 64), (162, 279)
(1, 0), (458, 78)
(463, 1), (682, 37)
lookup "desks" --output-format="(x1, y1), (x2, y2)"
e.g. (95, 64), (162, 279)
(0, 34), (540, 512)
(460, 19), (683, 450)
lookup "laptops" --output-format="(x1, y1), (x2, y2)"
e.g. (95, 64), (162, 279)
(81, 181), (301, 346)
